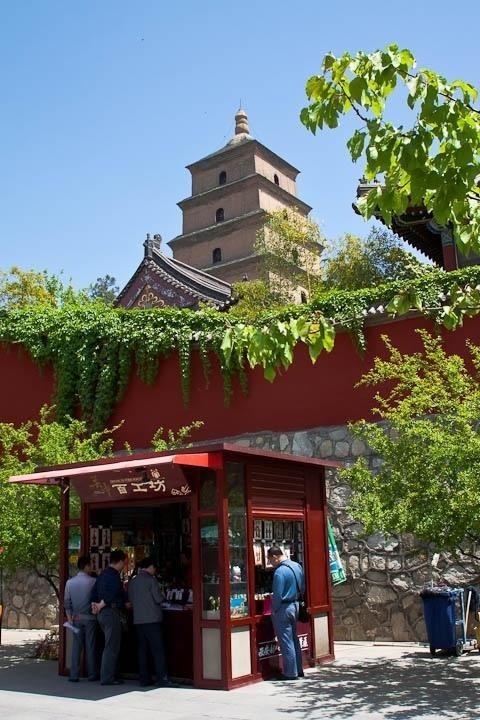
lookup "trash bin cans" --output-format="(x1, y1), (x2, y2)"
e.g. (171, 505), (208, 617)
(421, 587), (465, 656)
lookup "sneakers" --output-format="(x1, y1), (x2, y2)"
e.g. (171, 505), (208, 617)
(275, 672), (304, 680)
(101, 681), (125, 686)
(141, 679), (180, 688)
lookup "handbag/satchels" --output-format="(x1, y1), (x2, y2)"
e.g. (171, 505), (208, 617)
(298, 599), (310, 624)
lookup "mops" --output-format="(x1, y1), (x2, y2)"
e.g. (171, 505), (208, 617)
(464, 585), (480, 634)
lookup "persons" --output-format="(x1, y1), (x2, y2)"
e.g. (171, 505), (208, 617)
(266, 546), (305, 680)
(64, 556), (98, 682)
(180, 546), (193, 589)
(128, 557), (180, 687)
(91, 550), (127, 685)
